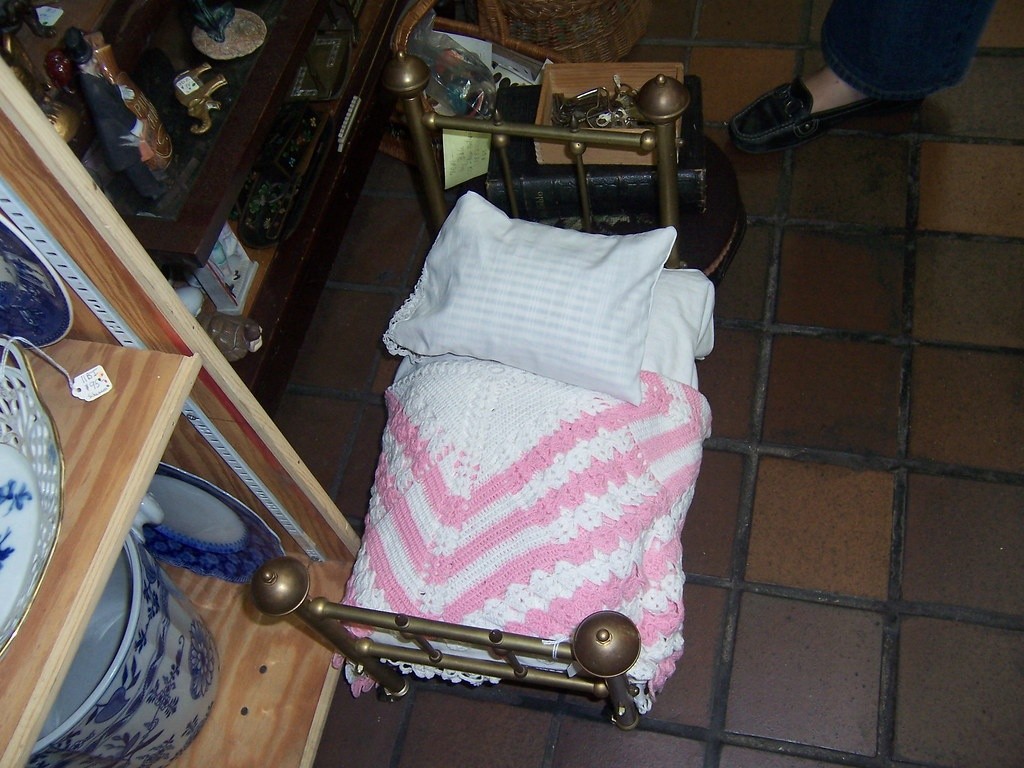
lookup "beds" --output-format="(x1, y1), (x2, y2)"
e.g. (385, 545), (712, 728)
(253, 54), (717, 728)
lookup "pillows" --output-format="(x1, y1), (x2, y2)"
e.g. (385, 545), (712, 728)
(383, 193), (676, 406)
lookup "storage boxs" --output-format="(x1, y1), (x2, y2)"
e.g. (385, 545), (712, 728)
(532, 62), (684, 166)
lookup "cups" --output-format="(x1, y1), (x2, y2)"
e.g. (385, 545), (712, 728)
(24, 531), (220, 768)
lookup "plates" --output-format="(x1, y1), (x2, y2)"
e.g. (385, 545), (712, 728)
(0, 214), (74, 350)
(138, 462), (287, 584)
(0, 443), (42, 630)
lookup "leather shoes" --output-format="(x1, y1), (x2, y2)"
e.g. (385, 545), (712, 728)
(727, 74), (926, 155)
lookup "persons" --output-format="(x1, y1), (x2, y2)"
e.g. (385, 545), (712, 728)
(727, 0), (997, 155)
(211, 240), (241, 281)
(62, 26), (172, 201)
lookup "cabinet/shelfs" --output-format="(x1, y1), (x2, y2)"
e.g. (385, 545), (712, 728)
(0, 55), (370, 767)
(0, 1), (402, 417)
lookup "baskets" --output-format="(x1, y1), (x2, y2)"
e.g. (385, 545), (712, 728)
(502, 0), (654, 64)
